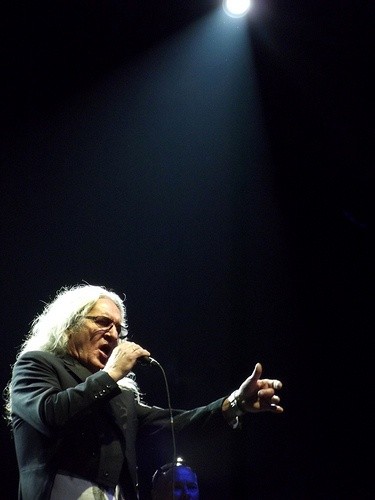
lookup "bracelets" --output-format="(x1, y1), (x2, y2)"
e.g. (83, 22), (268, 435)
(229, 391), (247, 416)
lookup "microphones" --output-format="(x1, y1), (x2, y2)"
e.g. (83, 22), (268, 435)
(113, 338), (155, 368)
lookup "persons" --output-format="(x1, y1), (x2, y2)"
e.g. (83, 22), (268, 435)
(10, 283), (286, 499)
(150, 461), (199, 500)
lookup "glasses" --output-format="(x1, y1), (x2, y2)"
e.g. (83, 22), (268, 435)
(83, 315), (128, 340)
